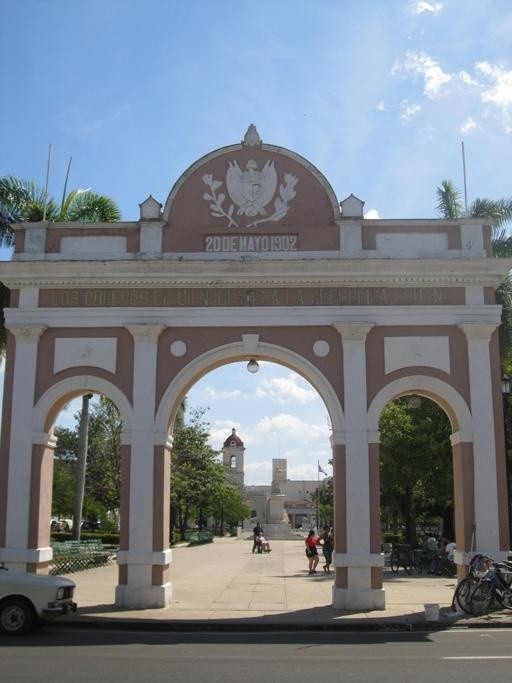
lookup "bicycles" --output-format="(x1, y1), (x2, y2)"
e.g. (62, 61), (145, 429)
(389, 541), (422, 576)
(451, 551), (511, 618)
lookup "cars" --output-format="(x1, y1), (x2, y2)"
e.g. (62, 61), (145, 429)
(51, 515), (104, 532)
(1, 564), (77, 635)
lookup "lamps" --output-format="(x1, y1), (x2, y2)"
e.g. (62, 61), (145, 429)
(247, 359), (259, 372)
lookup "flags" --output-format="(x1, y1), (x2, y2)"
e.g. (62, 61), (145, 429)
(319, 465), (327, 476)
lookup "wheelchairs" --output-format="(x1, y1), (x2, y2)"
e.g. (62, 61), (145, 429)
(255, 540), (272, 554)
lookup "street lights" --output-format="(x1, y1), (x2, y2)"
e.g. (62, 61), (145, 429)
(290, 502), (297, 528)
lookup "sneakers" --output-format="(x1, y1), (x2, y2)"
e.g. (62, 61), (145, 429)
(309, 570), (316, 574)
(323, 566), (326, 572)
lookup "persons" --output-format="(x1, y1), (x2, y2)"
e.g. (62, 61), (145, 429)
(304, 526), (457, 576)
(251, 521), (264, 554)
(256, 531), (272, 554)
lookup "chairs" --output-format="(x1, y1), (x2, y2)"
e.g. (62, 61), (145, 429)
(422, 544), (457, 576)
(49, 539), (114, 576)
